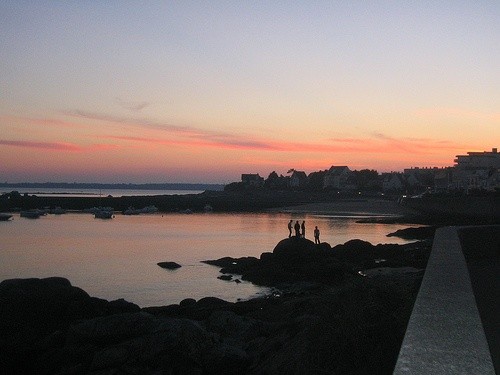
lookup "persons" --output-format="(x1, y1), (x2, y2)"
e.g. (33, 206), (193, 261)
(314, 226), (321, 245)
(301, 220), (305, 238)
(288, 219), (293, 237)
(295, 221), (300, 237)
